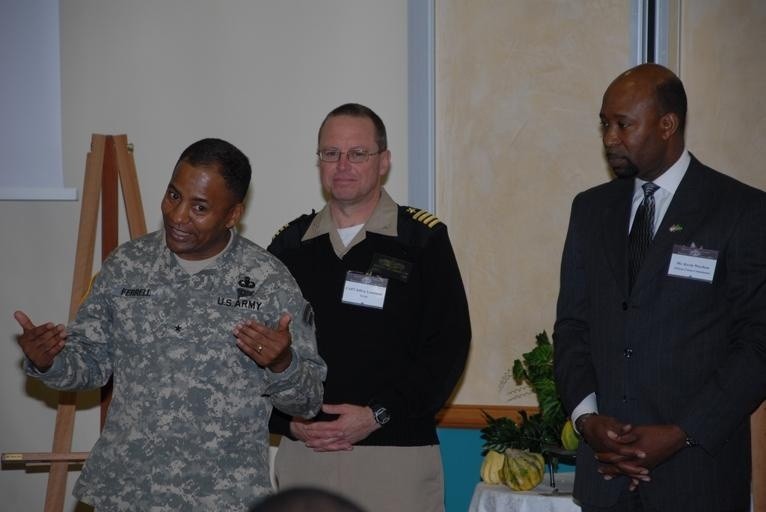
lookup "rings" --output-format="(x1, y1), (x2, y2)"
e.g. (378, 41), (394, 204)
(257, 344), (262, 352)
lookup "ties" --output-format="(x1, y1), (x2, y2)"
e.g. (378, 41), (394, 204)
(628, 181), (660, 299)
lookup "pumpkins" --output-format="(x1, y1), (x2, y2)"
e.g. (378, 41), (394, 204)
(502, 448), (545, 491)
(480, 451), (505, 485)
(561, 421), (579, 451)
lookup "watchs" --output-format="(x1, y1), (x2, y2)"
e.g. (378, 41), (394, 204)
(368, 399), (391, 426)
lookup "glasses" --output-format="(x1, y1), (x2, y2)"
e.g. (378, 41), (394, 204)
(316, 149), (381, 164)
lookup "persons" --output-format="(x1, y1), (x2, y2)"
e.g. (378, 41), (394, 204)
(12, 138), (329, 511)
(264, 103), (473, 512)
(552, 63), (766, 512)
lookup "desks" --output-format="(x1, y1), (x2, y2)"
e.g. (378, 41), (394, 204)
(466, 470), (587, 512)
